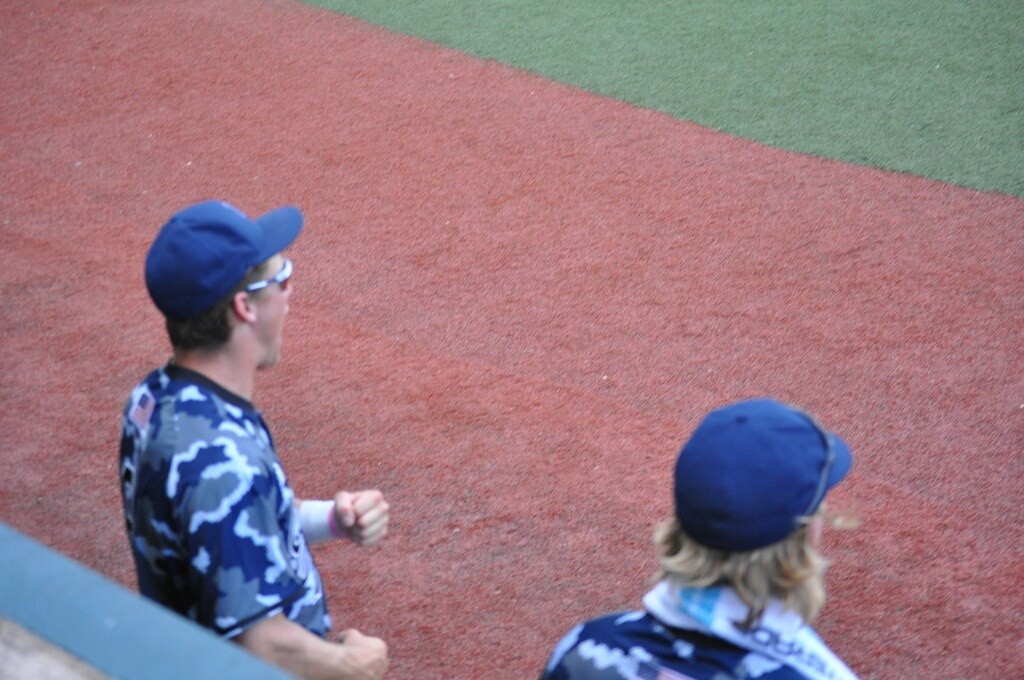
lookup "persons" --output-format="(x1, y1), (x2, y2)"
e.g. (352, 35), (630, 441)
(117, 199), (391, 678)
(541, 399), (866, 680)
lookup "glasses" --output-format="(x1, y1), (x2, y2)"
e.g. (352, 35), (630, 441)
(244, 259), (293, 292)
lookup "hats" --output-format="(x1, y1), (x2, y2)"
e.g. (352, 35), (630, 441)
(144, 199), (304, 319)
(675, 398), (852, 554)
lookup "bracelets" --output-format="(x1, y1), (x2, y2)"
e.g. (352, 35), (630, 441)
(299, 500), (343, 544)
